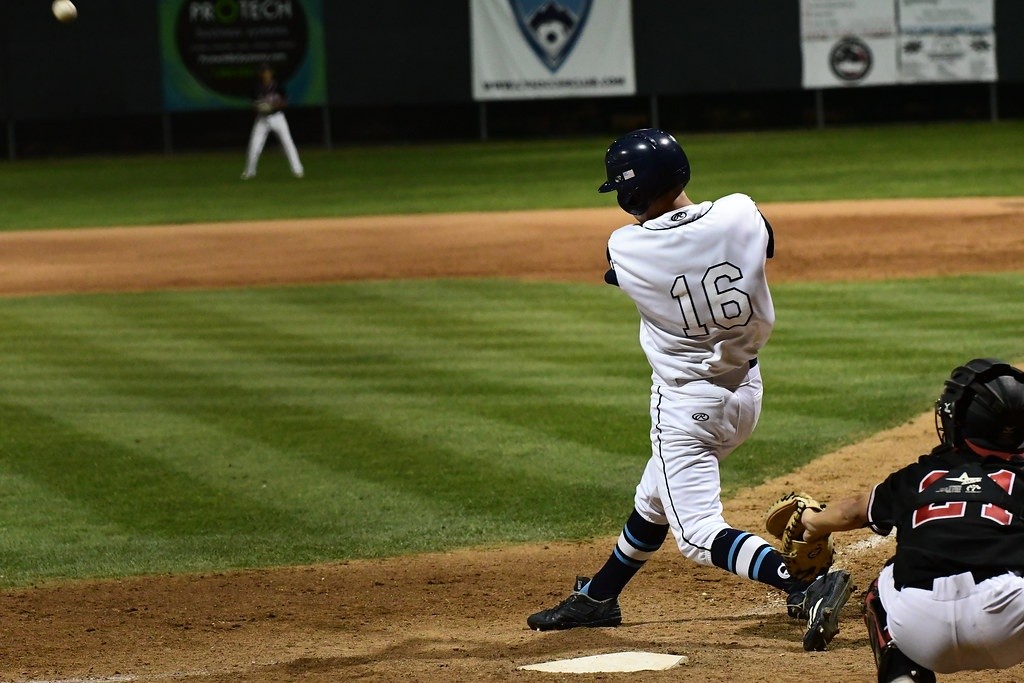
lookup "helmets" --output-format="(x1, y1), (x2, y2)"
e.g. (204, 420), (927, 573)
(934, 357), (1024, 471)
(597, 127), (690, 217)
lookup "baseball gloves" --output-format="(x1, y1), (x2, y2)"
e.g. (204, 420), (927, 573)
(766, 494), (837, 582)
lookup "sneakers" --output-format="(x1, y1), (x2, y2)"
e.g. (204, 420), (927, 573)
(786, 570), (858, 651)
(877, 642), (936, 683)
(527, 576), (623, 631)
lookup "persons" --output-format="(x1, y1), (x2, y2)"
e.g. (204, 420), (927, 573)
(526, 130), (856, 651)
(240, 68), (304, 181)
(801, 358), (1024, 683)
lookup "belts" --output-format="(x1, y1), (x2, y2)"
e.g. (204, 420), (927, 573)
(748, 357), (758, 369)
(903, 564), (1021, 591)
